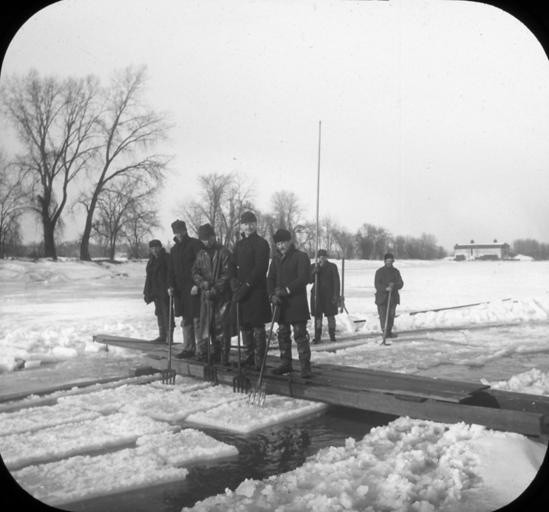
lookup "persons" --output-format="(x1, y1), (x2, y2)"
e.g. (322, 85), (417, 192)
(142, 238), (177, 344)
(190, 222), (234, 372)
(373, 253), (404, 338)
(164, 218), (207, 360)
(265, 228), (313, 378)
(227, 210), (274, 370)
(306, 248), (341, 344)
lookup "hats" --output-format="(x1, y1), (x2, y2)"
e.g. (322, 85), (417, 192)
(198, 223), (216, 241)
(149, 239), (162, 248)
(171, 220), (188, 234)
(384, 253), (394, 261)
(273, 229), (292, 243)
(317, 249), (330, 258)
(240, 211), (258, 224)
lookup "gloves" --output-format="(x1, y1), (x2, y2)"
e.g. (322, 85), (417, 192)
(270, 295), (286, 306)
(231, 283), (250, 302)
(270, 286), (289, 298)
(229, 277), (242, 292)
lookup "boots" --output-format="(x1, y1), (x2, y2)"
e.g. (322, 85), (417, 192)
(292, 328), (314, 378)
(234, 335), (254, 368)
(270, 329), (294, 375)
(254, 332), (267, 371)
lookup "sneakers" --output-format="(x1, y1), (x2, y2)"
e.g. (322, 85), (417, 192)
(174, 346), (232, 367)
(151, 335), (175, 345)
(382, 328), (398, 338)
(312, 327), (337, 344)
